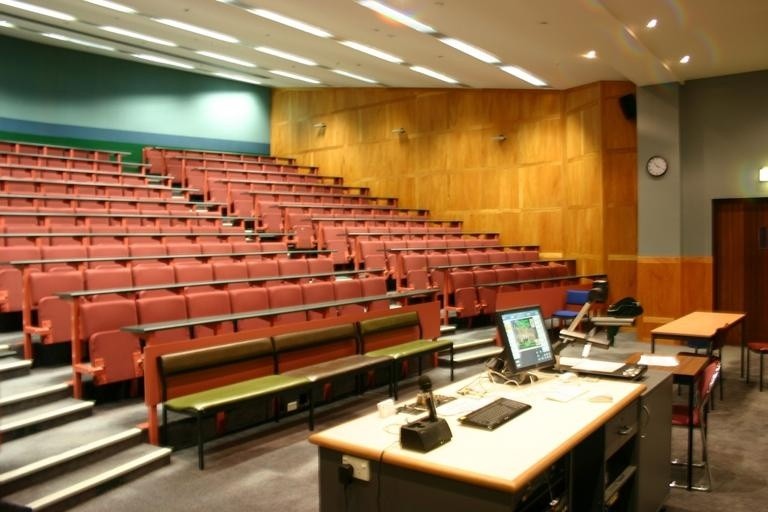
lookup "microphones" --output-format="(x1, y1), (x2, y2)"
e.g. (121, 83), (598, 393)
(417, 374), (437, 421)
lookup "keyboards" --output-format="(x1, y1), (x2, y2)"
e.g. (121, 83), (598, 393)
(458, 398), (531, 430)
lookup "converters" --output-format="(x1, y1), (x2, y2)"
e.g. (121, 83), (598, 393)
(337, 464), (353, 485)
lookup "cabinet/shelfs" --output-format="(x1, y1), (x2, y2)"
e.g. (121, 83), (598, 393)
(604, 370), (673, 511)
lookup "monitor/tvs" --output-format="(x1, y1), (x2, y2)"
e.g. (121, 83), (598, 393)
(497, 305), (557, 373)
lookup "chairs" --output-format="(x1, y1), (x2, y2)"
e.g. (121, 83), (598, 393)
(672, 359), (721, 491)
(745, 340), (768, 391)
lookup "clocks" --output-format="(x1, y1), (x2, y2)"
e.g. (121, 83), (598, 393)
(647, 155), (667, 178)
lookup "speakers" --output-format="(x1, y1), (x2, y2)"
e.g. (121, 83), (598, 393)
(620, 94), (637, 119)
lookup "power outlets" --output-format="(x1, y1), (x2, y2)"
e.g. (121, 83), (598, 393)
(341, 454), (371, 482)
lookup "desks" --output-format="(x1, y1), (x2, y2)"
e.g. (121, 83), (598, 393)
(307, 371), (646, 511)
(651, 311), (750, 411)
(623, 352), (711, 492)
